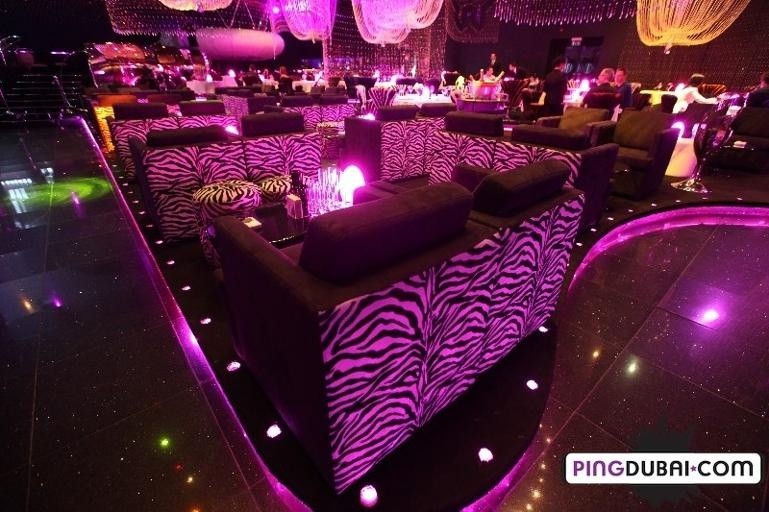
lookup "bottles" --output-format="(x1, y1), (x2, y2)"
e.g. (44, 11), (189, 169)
(288, 171), (307, 211)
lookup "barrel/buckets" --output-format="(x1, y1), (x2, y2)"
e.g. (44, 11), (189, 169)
(469, 80), (502, 101)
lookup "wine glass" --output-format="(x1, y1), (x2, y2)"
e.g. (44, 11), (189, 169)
(670, 113), (733, 195)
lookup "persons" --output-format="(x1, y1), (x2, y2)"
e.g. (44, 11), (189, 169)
(677, 73), (717, 104)
(743, 71), (769, 108)
(73, 50), (634, 118)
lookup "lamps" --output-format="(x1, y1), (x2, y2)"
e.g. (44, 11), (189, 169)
(105, 0), (753, 78)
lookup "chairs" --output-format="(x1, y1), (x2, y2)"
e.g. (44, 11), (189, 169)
(90, 66), (769, 504)
